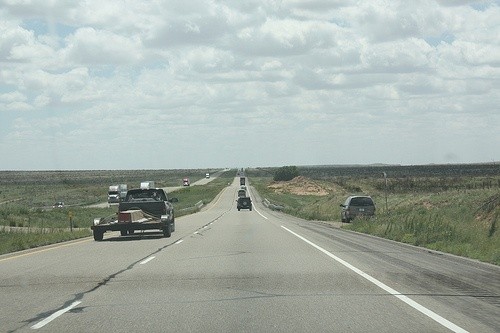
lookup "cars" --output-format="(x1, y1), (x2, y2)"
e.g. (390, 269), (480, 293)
(53, 202), (64, 208)
(241, 186), (246, 190)
(238, 190), (245, 196)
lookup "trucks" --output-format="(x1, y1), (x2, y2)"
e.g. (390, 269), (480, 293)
(240, 178), (245, 185)
(90, 188), (178, 241)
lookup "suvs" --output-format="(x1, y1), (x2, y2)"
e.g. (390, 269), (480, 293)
(236, 196), (252, 211)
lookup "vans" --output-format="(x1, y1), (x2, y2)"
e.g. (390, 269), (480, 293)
(339, 195), (376, 223)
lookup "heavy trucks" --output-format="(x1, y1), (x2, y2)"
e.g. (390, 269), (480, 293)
(205, 173), (209, 178)
(140, 181), (154, 188)
(183, 179), (189, 185)
(107, 184), (127, 202)
